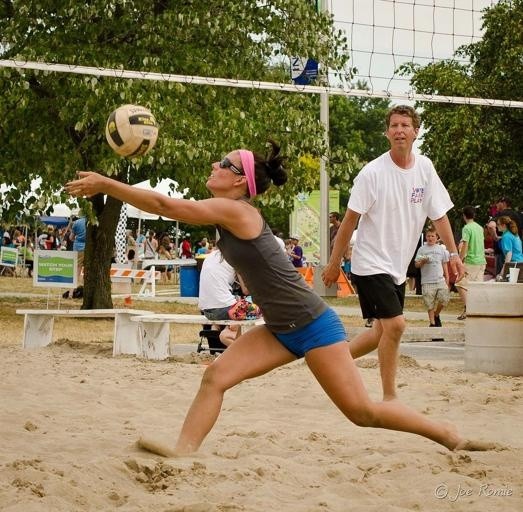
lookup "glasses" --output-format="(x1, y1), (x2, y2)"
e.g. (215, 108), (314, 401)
(220, 159), (243, 176)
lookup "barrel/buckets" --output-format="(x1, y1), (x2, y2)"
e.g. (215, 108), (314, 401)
(179, 266), (198, 296)
(504, 261), (523, 283)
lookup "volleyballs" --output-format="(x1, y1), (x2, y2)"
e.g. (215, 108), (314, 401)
(106, 105), (159, 157)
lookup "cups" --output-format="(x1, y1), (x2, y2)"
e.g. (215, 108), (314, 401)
(508, 267), (519, 283)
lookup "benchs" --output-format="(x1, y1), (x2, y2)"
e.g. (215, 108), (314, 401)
(130, 313), (266, 360)
(16, 308), (156, 356)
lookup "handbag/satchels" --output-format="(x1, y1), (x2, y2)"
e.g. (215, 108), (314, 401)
(228, 301), (262, 320)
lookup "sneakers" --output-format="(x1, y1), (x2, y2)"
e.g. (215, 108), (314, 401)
(365, 319), (373, 327)
(457, 306), (466, 320)
(430, 323), (435, 327)
(434, 311), (442, 327)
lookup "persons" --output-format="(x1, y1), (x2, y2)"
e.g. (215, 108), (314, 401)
(1, 195), (523, 354)
(324, 105), (465, 408)
(64, 138), (466, 459)
